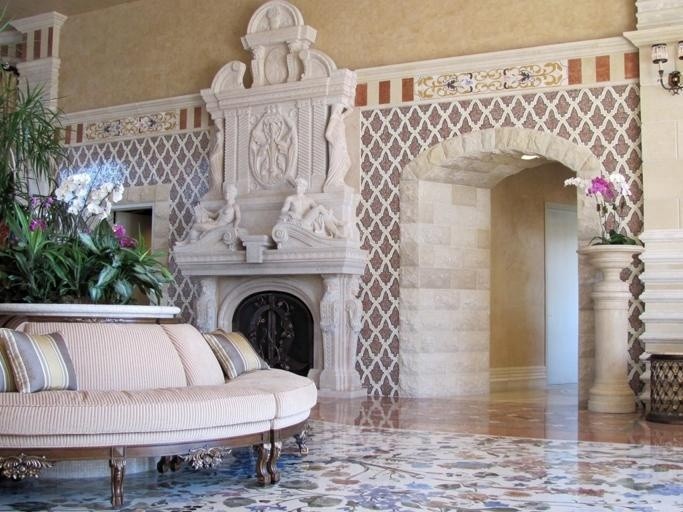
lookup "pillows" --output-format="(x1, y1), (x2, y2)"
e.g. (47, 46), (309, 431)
(0, 327), (77, 393)
(200, 330), (271, 381)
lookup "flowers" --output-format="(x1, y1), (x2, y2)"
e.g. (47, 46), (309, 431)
(18, 173), (140, 267)
(563, 170), (637, 247)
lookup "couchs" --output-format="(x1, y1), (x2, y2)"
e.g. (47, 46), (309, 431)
(0, 319), (318, 507)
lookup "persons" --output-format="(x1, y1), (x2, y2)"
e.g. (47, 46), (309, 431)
(280, 178), (345, 239)
(175, 183), (241, 247)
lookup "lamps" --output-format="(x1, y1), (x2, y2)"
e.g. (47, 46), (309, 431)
(651, 41), (682, 96)
